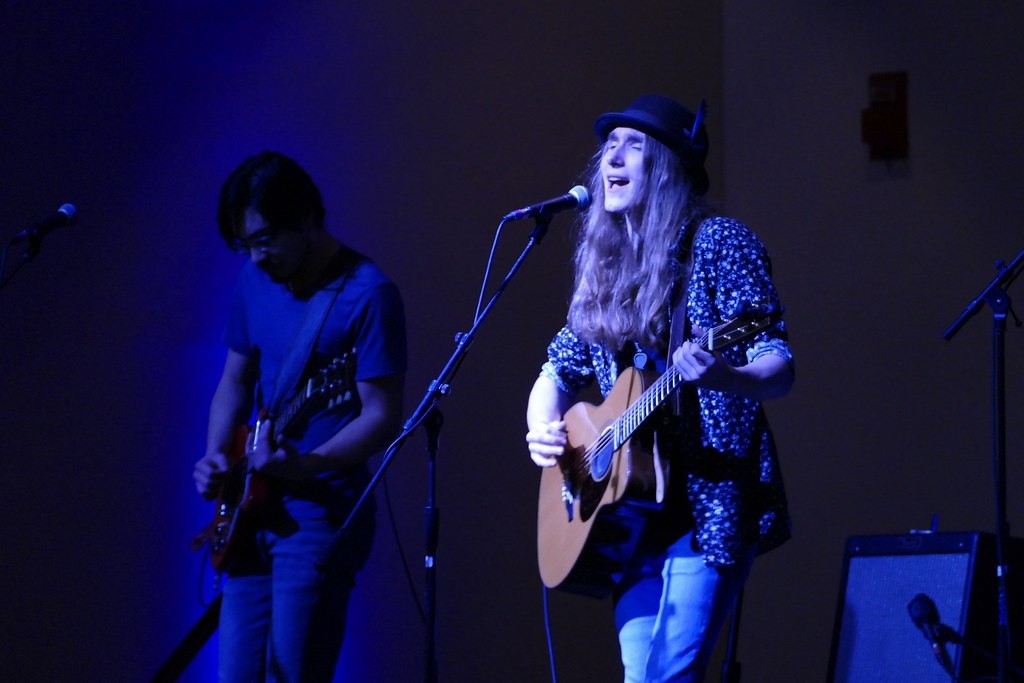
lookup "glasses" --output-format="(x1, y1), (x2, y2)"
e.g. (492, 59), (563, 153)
(229, 224), (291, 258)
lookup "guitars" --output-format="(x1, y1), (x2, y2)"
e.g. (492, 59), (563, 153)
(209, 345), (360, 573)
(536, 304), (788, 601)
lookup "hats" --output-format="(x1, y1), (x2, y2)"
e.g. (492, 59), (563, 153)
(593, 91), (710, 199)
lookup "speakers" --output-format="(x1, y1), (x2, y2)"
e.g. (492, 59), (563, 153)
(827, 530), (1023, 683)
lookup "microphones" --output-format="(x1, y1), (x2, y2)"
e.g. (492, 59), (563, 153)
(504, 183), (592, 225)
(10, 201), (78, 248)
(908, 593), (951, 670)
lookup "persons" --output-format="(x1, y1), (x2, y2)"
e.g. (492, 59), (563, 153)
(194, 150), (410, 683)
(526, 92), (799, 683)
